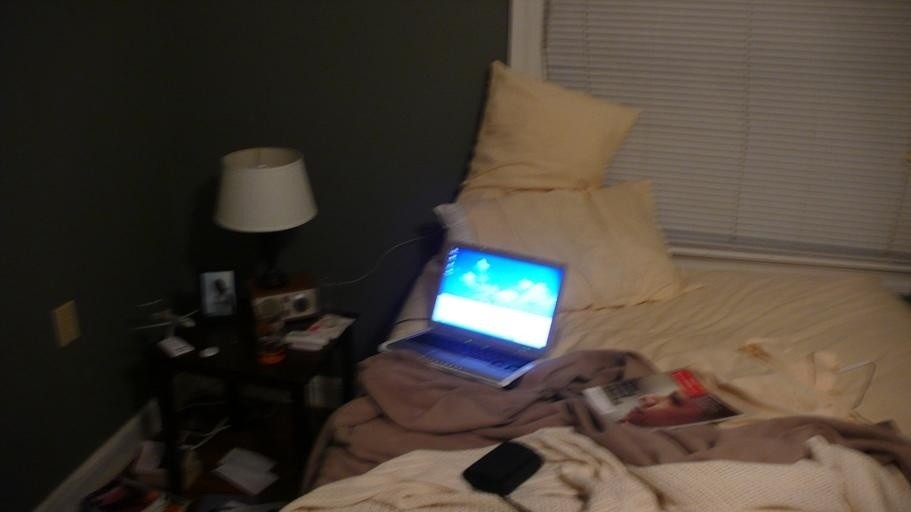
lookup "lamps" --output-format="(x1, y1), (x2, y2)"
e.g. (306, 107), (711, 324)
(216, 145), (318, 289)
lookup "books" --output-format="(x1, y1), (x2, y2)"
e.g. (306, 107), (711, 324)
(579, 365), (744, 433)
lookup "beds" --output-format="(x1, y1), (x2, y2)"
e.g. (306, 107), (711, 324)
(281, 254), (911, 512)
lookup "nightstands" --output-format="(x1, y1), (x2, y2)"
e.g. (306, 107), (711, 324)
(156, 300), (363, 503)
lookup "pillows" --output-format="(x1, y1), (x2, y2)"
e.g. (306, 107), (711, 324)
(465, 59), (645, 194)
(436, 176), (706, 314)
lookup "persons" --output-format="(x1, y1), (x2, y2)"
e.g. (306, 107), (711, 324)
(206, 275), (237, 316)
(623, 385), (736, 428)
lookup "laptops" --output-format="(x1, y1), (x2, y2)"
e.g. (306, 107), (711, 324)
(376, 242), (569, 391)
